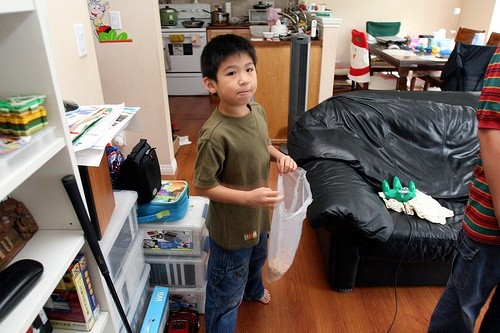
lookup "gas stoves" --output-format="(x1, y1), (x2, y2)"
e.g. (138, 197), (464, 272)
(159, 3), (212, 33)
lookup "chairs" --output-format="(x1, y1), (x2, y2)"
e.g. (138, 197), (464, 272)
(454, 27), (487, 46)
(348, 29), (399, 92)
(409, 44), (497, 91)
(366, 21), (402, 75)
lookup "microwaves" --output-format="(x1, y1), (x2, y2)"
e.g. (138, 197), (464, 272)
(249, 8), (281, 24)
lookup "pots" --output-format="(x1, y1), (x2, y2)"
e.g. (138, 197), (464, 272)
(210, 7), (229, 24)
(253, 2), (273, 9)
(160, 6), (180, 26)
(181, 17), (204, 28)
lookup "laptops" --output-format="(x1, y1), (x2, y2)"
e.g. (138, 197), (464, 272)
(376, 36), (407, 44)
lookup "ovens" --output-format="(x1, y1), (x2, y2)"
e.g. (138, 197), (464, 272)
(162, 32), (210, 96)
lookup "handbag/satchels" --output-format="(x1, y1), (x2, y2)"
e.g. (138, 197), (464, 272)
(120, 139), (162, 203)
(266, 167), (313, 283)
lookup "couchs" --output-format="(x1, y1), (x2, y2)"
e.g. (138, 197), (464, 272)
(284, 90), (487, 291)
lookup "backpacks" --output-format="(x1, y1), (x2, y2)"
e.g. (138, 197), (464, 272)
(137, 178), (190, 223)
(106, 142), (124, 189)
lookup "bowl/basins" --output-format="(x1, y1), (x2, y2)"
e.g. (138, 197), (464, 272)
(250, 26), (270, 36)
(263, 32), (275, 38)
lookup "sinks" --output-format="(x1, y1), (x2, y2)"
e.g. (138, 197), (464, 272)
(279, 29), (298, 39)
(286, 25), (296, 30)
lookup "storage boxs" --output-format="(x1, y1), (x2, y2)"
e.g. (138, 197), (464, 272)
(97, 178), (208, 333)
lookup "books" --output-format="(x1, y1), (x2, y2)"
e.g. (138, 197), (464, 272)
(25, 252), (101, 333)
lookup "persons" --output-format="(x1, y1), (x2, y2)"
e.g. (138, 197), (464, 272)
(426, 38), (500, 333)
(192, 34), (297, 333)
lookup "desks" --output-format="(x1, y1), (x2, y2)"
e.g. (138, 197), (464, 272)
(368, 40), (447, 91)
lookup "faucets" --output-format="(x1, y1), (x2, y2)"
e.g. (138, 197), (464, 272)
(277, 12), (299, 28)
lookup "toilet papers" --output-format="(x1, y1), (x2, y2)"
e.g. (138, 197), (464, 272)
(225, 2), (231, 22)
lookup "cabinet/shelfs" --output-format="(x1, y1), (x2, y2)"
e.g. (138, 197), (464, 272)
(0, 0), (117, 333)
(206, 25), (322, 145)
